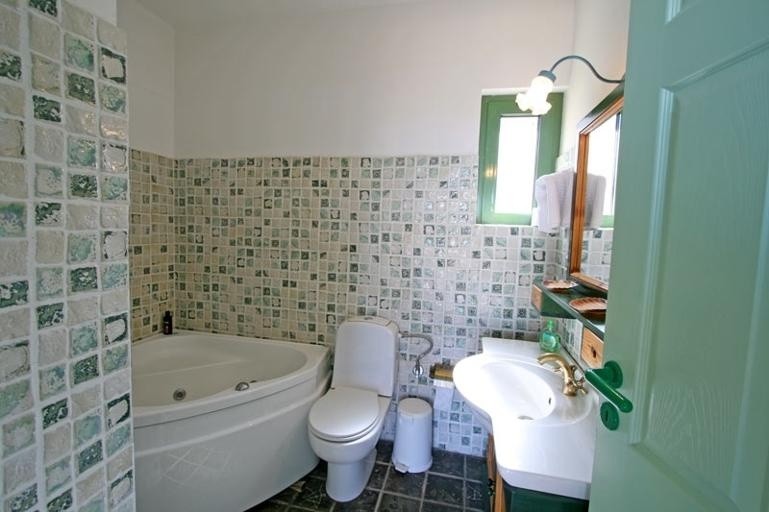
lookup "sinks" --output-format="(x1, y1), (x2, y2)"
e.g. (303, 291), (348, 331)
(452, 354), (592, 425)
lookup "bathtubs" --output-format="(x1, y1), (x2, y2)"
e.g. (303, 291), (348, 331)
(132, 329), (332, 512)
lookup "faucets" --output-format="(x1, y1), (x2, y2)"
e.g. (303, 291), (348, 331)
(538, 352), (577, 397)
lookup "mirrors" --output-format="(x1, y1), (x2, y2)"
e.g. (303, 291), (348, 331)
(565, 80), (623, 298)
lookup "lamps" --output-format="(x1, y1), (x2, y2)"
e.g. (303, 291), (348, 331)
(515, 52), (625, 119)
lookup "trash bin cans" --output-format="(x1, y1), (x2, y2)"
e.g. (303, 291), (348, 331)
(391, 398), (433, 474)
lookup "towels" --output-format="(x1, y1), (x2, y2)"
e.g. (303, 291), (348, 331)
(533, 169), (573, 234)
(583, 173), (606, 230)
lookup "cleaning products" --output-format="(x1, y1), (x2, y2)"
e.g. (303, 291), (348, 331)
(163, 311), (172, 334)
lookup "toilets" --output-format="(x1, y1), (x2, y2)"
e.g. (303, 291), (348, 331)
(308, 316), (399, 503)
